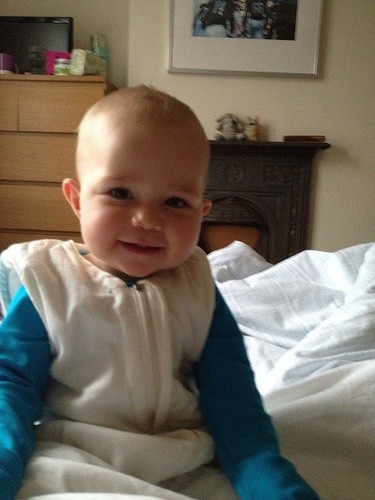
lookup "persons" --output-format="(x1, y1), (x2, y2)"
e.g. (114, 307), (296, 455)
(0, 85), (321, 500)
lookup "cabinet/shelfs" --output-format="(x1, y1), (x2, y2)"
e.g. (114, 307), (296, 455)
(0, 73), (120, 254)
(196, 140), (332, 265)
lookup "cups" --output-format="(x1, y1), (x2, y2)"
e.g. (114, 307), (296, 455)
(0, 54), (16, 74)
(90, 34), (109, 60)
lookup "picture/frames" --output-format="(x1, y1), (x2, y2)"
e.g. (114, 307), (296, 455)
(167, 0), (326, 79)
